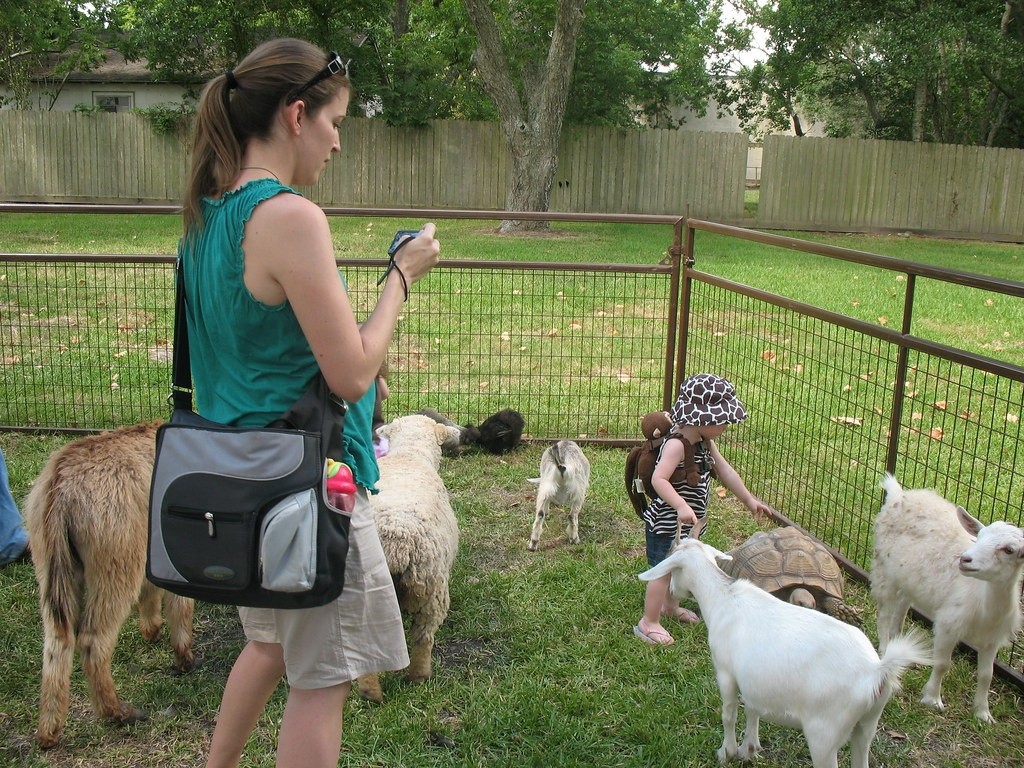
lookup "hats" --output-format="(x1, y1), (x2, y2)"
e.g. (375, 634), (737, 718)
(672, 373), (748, 427)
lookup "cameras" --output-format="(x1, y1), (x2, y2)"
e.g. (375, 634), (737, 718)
(388, 230), (420, 256)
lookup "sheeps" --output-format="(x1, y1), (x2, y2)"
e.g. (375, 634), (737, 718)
(20, 411), (205, 746)
(524, 440), (591, 552)
(635, 513), (948, 768)
(870, 470), (1024, 726)
(340, 416), (461, 704)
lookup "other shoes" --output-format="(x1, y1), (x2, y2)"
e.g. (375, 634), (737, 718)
(0, 537), (32, 568)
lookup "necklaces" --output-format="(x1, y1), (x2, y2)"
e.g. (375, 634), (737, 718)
(241, 167), (280, 180)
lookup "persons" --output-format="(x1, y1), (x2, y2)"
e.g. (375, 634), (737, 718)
(179, 37), (443, 768)
(634, 373), (772, 647)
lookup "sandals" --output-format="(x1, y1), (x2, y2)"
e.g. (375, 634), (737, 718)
(634, 617), (675, 645)
(659, 607), (700, 625)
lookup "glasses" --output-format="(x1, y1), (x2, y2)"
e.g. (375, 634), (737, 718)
(285, 49), (348, 104)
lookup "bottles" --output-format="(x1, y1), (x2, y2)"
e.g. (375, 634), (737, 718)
(324, 458), (356, 514)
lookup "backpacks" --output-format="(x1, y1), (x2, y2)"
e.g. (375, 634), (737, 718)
(624, 412), (718, 521)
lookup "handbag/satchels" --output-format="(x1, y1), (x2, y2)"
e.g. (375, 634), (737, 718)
(145, 406), (354, 610)
